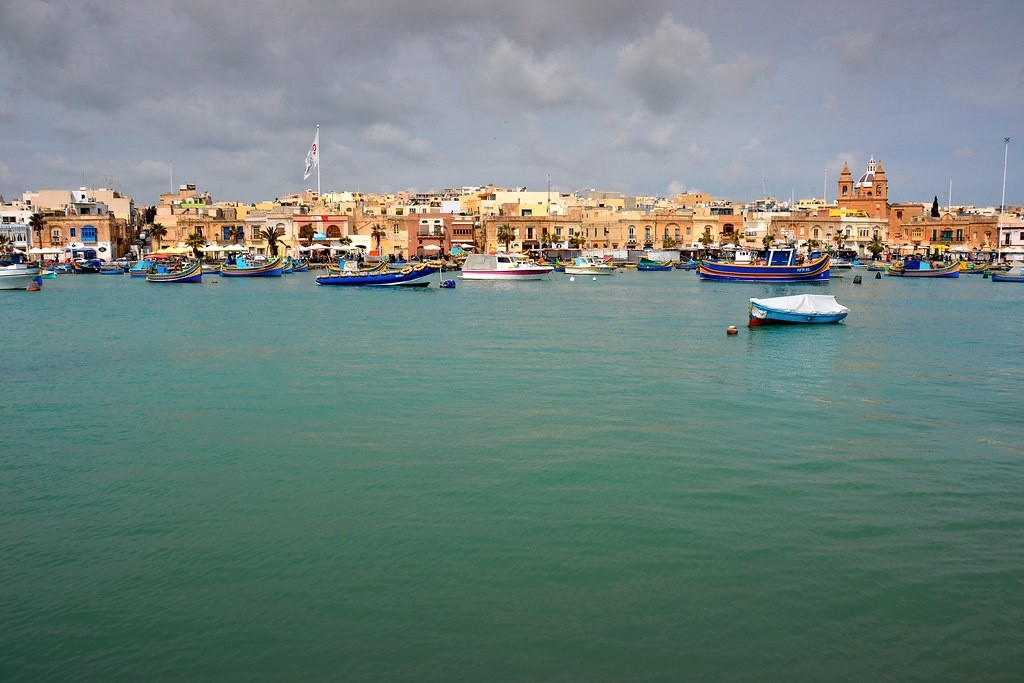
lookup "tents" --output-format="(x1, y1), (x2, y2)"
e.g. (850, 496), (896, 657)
(424, 244), (441, 255)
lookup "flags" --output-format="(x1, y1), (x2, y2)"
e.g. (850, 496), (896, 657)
(304, 132), (318, 180)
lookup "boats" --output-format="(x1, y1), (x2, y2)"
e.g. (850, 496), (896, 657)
(674, 262), (699, 269)
(698, 253), (832, 285)
(991, 260), (1024, 282)
(564, 256), (618, 276)
(97, 262), (126, 275)
(866, 261), (888, 271)
(457, 253), (557, 280)
(145, 261), (203, 282)
(852, 260), (865, 267)
(313, 258), (445, 287)
(0, 247), (107, 291)
(953, 257), (990, 273)
(123, 263), (130, 270)
(826, 255), (854, 268)
(746, 293), (849, 326)
(127, 246), (310, 277)
(886, 257), (963, 278)
(637, 255), (675, 271)
(441, 259), (464, 272)
(325, 259), (389, 272)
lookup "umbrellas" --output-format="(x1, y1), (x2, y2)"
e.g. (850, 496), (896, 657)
(462, 243), (475, 249)
(153, 244), (247, 260)
(935, 245), (969, 257)
(28, 247), (65, 254)
(889, 245), (930, 254)
(294, 243), (357, 257)
